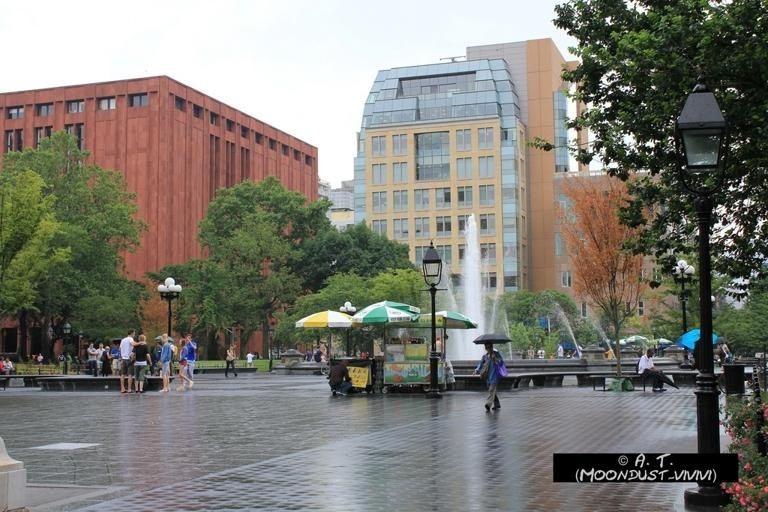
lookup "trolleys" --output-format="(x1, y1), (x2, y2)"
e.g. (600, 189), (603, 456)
(327, 355), (383, 394)
(379, 359), (447, 394)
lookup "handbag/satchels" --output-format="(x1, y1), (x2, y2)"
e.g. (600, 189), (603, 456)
(496, 361), (508, 378)
(100, 356), (105, 362)
(480, 364), (488, 379)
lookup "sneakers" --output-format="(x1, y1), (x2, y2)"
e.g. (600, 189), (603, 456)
(332, 390), (336, 396)
(337, 393), (343, 396)
(176, 387), (185, 392)
(188, 381), (194, 389)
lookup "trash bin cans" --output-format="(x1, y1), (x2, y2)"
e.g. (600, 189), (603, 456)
(721, 364), (747, 396)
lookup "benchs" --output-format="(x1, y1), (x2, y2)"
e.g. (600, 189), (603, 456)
(584, 374), (650, 392)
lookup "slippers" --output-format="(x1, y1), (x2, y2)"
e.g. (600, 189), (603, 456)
(121, 389), (134, 393)
(136, 390), (146, 393)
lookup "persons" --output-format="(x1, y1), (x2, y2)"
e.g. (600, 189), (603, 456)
(473, 342), (503, 412)
(328, 362), (352, 397)
(527, 345), (534, 359)
(537, 347), (545, 359)
(436, 336), (441, 352)
(247, 352), (255, 367)
(605, 346), (613, 358)
(313, 346), (326, 363)
(722, 344), (729, 352)
(553, 343), (563, 359)
(638, 348), (666, 392)
(0, 328), (197, 394)
(224, 345), (237, 377)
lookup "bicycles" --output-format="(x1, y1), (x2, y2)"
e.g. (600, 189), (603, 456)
(321, 365), (330, 376)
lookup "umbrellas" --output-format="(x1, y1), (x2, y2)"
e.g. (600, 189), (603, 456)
(624, 334), (647, 345)
(428, 309), (478, 356)
(351, 299), (420, 344)
(653, 370), (679, 389)
(472, 334), (514, 351)
(647, 337), (672, 347)
(675, 329), (717, 351)
(611, 338), (627, 347)
(294, 309), (362, 360)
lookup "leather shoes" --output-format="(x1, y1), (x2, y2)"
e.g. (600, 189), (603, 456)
(485, 403), (491, 411)
(660, 388), (667, 391)
(492, 405), (501, 409)
(652, 389), (660, 392)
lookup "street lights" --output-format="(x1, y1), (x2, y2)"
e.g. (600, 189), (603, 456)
(156, 275), (183, 338)
(670, 67), (743, 511)
(422, 235), (448, 400)
(61, 321), (73, 375)
(338, 300), (356, 358)
(757, 334), (767, 392)
(669, 258), (696, 369)
(697, 295), (715, 308)
(46, 326), (54, 365)
(78, 330), (84, 363)
